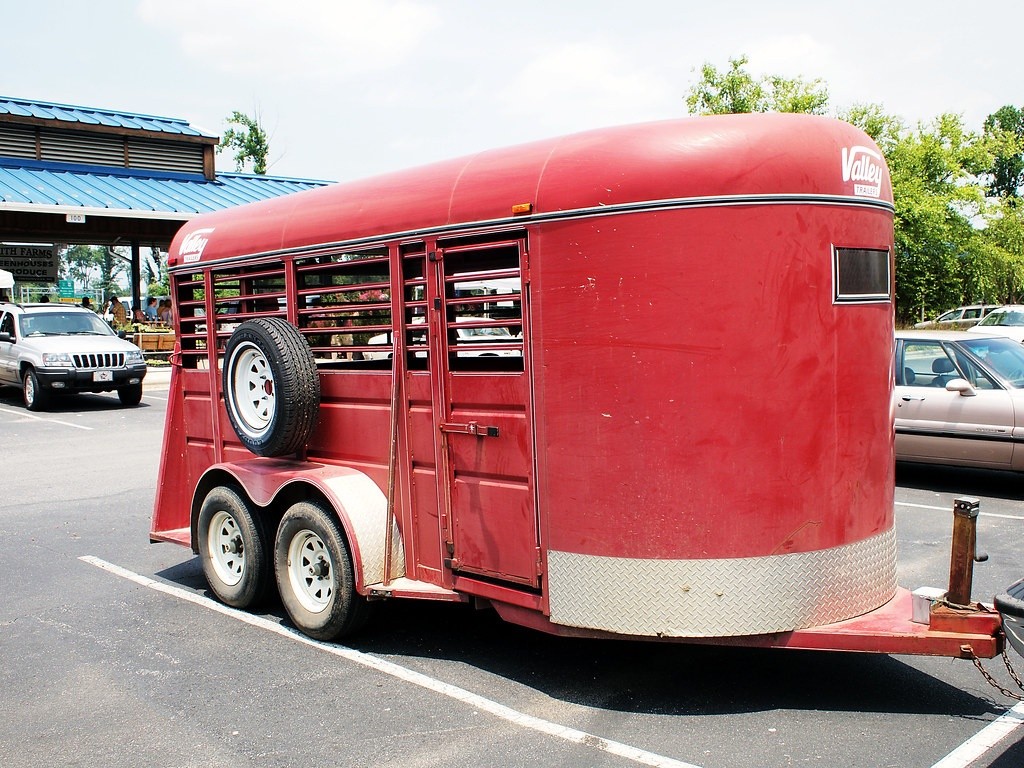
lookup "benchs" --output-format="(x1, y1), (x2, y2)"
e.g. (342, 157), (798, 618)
(133, 333), (177, 356)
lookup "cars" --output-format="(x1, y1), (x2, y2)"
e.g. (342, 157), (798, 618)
(968, 305), (1024, 346)
(892, 329), (1024, 482)
(368, 316), (522, 359)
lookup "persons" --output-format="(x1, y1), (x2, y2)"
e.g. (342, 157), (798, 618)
(131, 306), (144, 323)
(160, 299), (172, 327)
(80, 297), (94, 311)
(157, 300), (166, 317)
(329, 292), (359, 361)
(109, 296), (127, 325)
(145, 298), (158, 321)
(39, 296), (49, 303)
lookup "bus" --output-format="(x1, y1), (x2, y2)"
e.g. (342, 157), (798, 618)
(410, 265), (521, 323)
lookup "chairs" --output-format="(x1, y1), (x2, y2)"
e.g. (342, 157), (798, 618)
(931, 357), (961, 387)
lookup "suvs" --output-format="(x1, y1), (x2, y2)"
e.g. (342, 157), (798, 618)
(0, 301), (148, 412)
(910, 306), (1001, 332)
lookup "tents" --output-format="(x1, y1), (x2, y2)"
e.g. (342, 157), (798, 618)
(415, 268), (521, 318)
